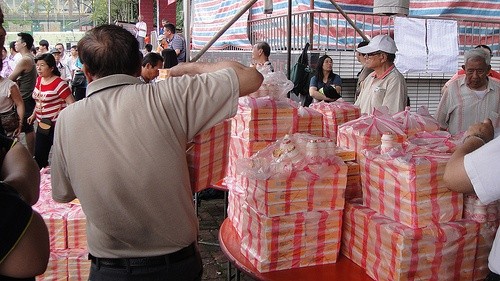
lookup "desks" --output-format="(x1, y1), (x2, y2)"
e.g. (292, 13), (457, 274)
(195, 180), (229, 247)
(218, 217), (375, 281)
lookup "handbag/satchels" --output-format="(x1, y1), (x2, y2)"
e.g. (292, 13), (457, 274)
(72, 73), (86, 87)
(0, 112), (19, 132)
(37, 119), (52, 135)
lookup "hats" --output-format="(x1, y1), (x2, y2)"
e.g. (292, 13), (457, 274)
(356, 34), (397, 54)
(50, 48), (61, 55)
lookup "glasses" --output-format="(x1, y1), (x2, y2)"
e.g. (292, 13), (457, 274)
(366, 51), (380, 58)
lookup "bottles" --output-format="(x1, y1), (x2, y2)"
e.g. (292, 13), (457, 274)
(474, 197), (488, 223)
(284, 139), (308, 170)
(327, 139), (336, 165)
(381, 130), (394, 156)
(306, 140), (319, 166)
(488, 199), (500, 222)
(286, 132), (329, 158)
(463, 194), (476, 219)
(317, 139), (328, 165)
(248, 63), (273, 98)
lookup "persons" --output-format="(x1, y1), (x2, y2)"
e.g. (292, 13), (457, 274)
(118, 14), (186, 84)
(434, 45), (500, 135)
(0, 6), (89, 281)
(445, 118), (500, 281)
(309, 55), (342, 103)
(250, 42), (274, 75)
(354, 35), (410, 114)
(51, 24), (264, 281)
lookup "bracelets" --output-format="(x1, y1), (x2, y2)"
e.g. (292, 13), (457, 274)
(462, 134), (487, 144)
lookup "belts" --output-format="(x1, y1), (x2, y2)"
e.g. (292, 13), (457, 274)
(91, 243), (198, 268)
